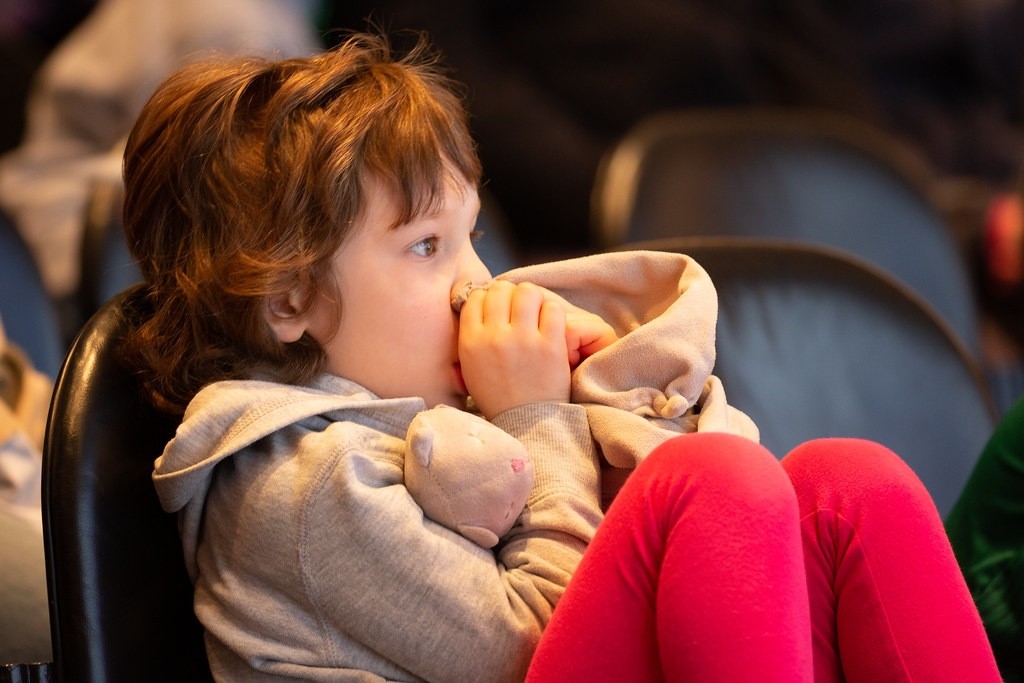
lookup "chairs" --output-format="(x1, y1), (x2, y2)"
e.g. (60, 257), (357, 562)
(630, 234), (1000, 519)
(604, 107), (986, 367)
(43, 284), (239, 683)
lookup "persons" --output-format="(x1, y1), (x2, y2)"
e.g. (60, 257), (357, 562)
(0, 332), (53, 665)
(120, 30), (1004, 683)
(0, 0), (322, 358)
